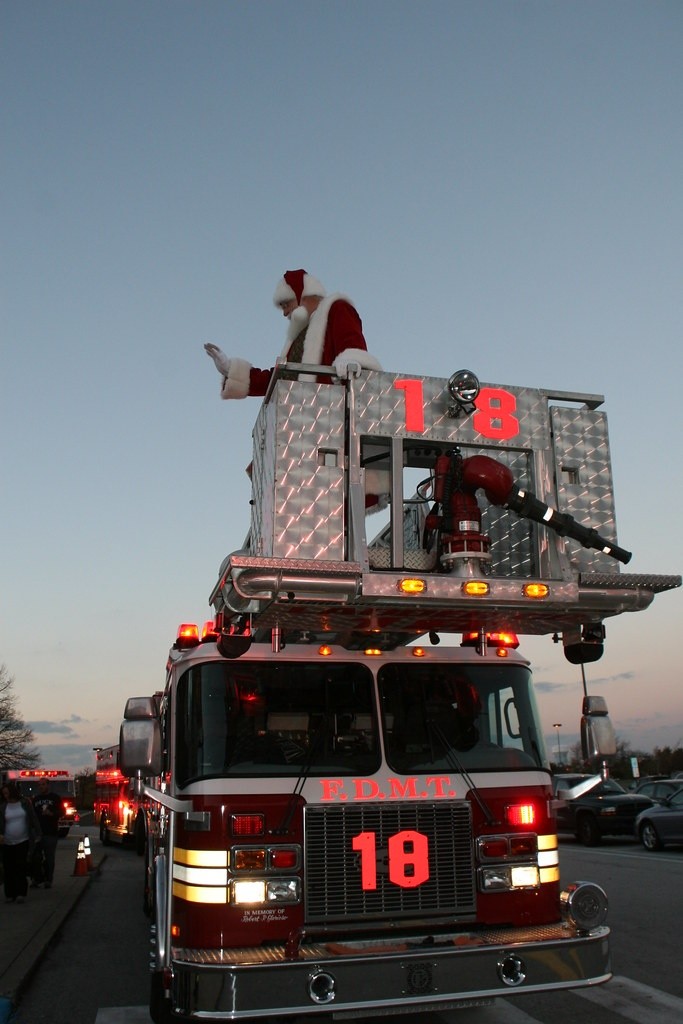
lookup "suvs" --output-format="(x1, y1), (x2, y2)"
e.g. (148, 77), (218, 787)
(552, 773), (653, 846)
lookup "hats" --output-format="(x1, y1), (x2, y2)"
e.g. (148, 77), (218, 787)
(275, 269), (325, 327)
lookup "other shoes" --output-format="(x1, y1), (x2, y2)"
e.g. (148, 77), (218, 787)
(6, 898), (12, 903)
(43, 881), (51, 889)
(16, 896), (24, 904)
(29, 879), (40, 888)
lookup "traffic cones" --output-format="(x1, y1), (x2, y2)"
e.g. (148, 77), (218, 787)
(83, 833), (99, 872)
(70, 835), (93, 877)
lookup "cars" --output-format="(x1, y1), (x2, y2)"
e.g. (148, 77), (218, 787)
(634, 787), (683, 852)
(627, 768), (683, 804)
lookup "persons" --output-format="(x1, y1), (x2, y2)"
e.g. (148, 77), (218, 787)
(1, 774), (62, 903)
(203, 266), (379, 401)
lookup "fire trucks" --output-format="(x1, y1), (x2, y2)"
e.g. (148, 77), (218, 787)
(1, 769), (80, 839)
(93, 355), (682, 1022)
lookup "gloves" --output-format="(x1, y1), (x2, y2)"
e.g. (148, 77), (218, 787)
(204, 344), (232, 377)
(331, 359), (362, 382)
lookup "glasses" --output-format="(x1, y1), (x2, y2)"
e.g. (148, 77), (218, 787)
(37, 782), (46, 786)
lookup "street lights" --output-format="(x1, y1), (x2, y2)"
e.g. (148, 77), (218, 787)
(552, 724), (563, 769)
